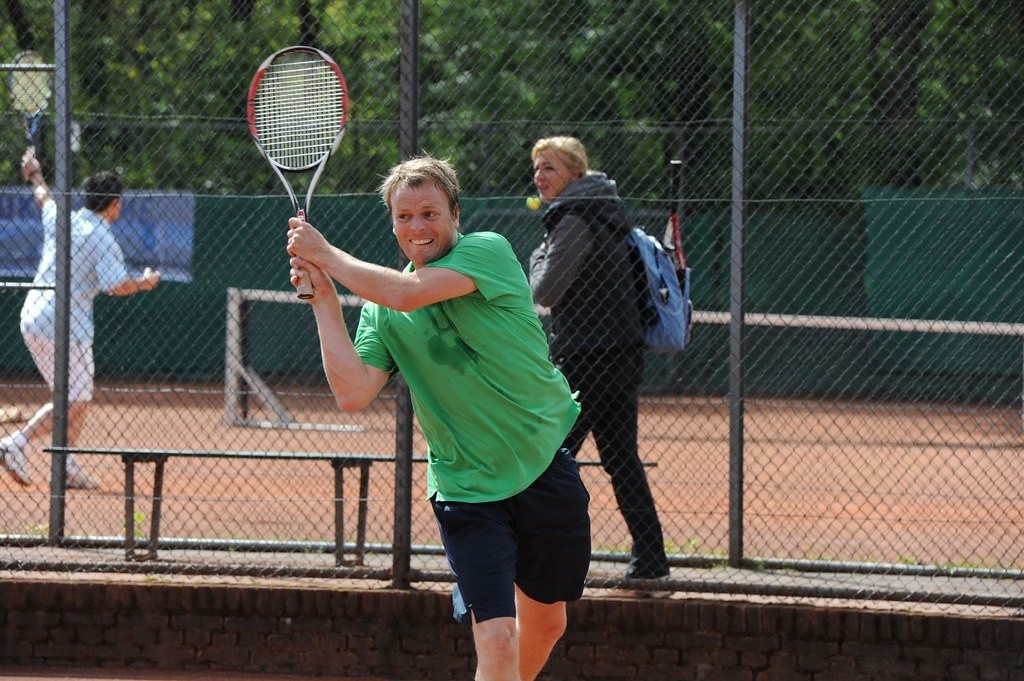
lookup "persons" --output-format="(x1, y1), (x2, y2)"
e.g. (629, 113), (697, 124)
(0, 144), (160, 486)
(525, 137), (672, 587)
(287, 149), (591, 680)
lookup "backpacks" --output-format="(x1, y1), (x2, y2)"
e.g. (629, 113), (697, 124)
(625, 223), (694, 354)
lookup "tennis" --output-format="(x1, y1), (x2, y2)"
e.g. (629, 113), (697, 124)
(526, 196), (544, 210)
(8, 50), (55, 149)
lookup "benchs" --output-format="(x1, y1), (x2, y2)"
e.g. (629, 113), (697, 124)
(42, 445), (658, 572)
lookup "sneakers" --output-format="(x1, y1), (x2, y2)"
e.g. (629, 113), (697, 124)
(625, 565), (672, 582)
(47, 465), (99, 489)
(1, 436), (31, 485)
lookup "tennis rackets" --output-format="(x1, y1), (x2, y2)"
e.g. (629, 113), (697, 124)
(243, 46), (352, 300)
